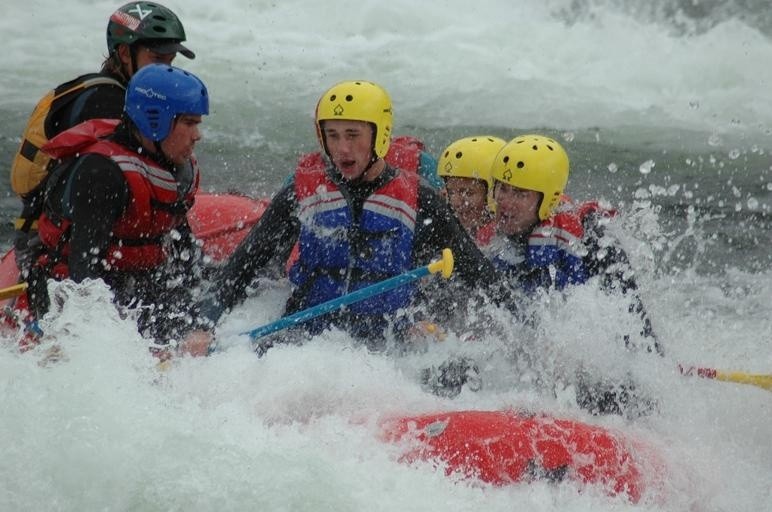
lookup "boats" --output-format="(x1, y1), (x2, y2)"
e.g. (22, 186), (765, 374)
(1, 189), (664, 507)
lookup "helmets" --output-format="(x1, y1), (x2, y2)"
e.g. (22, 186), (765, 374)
(105, 1), (195, 60)
(438, 135), (569, 221)
(124, 65), (209, 141)
(315, 79), (392, 159)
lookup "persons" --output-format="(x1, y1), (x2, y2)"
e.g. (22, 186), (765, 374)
(272, 136), (445, 280)
(12, 0), (194, 270)
(419, 133), (662, 414)
(176, 82), (517, 365)
(24, 60), (217, 346)
